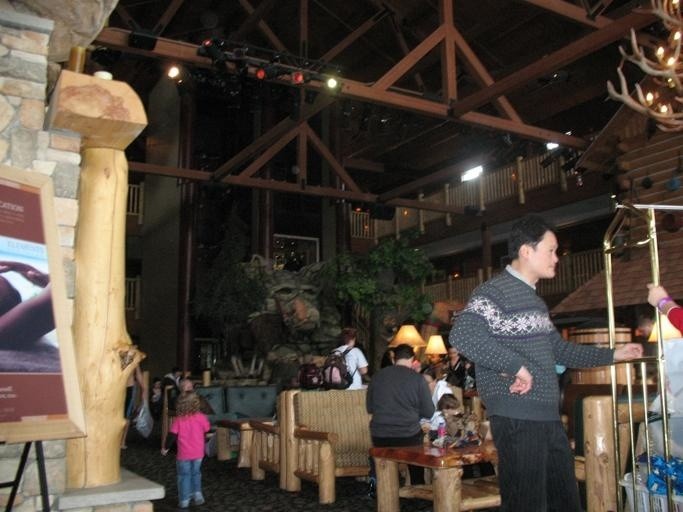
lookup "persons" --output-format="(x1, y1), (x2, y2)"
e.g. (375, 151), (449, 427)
(168, 392), (211, 508)
(161, 379), (215, 456)
(164, 368), (183, 385)
(367, 324), (487, 498)
(178, 368), (192, 383)
(646, 281), (683, 336)
(332, 327), (369, 390)
(150, 377), (166, 420)
(122, 351), (147, 450)
(0, 259), (56, 347)
(450, 218), (643, 511)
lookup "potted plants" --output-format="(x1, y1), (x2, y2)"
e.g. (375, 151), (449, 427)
(0, 165), (87, 443)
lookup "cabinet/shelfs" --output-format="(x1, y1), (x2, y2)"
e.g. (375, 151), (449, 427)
(438, 423), (445, 438)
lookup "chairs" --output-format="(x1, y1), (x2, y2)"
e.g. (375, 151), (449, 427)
(180, 491), (204, 508)
(368, 478), (377, 499)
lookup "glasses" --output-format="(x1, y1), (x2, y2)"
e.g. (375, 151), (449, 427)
(603, 1), (683, 132)
(387, 324), (448, 362)
(199, 39), (340, 91)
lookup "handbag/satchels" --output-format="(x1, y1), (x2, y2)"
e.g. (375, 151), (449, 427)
(658, 297), (672, 311)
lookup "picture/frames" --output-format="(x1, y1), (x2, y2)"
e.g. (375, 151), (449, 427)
(215, 419), (253, 469)
(371, 441), (502, 512)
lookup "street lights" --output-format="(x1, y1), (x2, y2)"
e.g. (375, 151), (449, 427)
(446, 413), (482, 448)
(323, 348), (357, 388)
(299, 364), (322, 388)
(133, 399), (154, 438)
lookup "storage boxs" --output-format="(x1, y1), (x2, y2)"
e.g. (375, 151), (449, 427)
(251, 387), (373, 504)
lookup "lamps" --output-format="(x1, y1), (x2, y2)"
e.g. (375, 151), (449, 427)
(194, 386), (282, 426)
